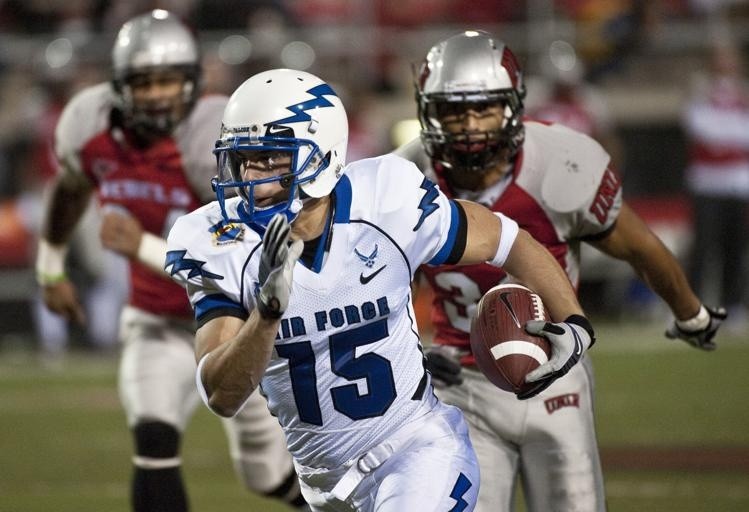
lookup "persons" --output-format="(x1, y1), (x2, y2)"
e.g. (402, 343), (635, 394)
(389, 29), (730, 511)
(161, 67), (597, 511)
(32, 6), (309, 511)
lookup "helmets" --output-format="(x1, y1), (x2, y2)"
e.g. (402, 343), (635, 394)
(113, 10), (201, 82)
(220, 68), (350, 200)
(412, 31), (524, 147)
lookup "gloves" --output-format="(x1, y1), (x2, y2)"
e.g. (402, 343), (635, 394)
(518, 315), (596, 400)
(254, 213), (305, 319)
(421, 346), (464, 389)
(665, 306), (727, 350)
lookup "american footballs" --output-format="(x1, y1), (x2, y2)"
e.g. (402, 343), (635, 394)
(469, 282), (552, 394)
